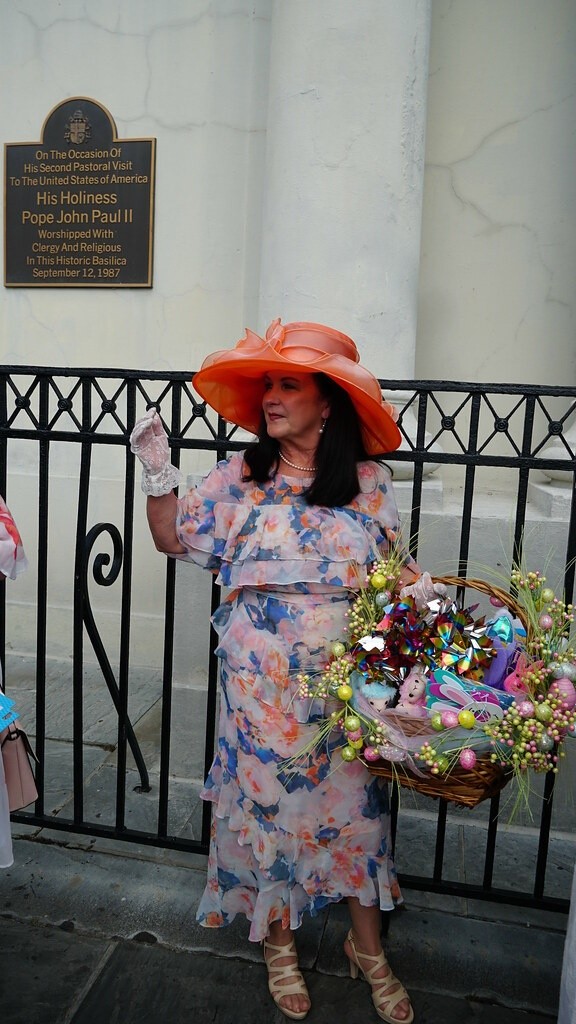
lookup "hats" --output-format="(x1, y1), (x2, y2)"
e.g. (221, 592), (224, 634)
(191, 317), (402, 455)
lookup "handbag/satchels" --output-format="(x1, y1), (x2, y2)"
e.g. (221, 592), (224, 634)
(0, 713), (42, 813)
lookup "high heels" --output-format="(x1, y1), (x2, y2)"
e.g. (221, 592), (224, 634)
(259, 937), (310, 1020)
(347, 927), (415, 1024)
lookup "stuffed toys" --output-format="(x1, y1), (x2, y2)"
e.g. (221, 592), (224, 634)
(502, 653), (545, 696)
(356, 663), (430, 718)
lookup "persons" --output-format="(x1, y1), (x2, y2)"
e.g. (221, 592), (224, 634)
(129, 321), (425, 1024)
(1, 495), (28, 869)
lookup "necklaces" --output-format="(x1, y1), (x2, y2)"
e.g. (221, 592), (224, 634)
(279, 452), (317, 473)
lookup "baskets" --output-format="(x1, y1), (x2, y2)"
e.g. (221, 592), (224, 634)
(347, 575), (549, 808)
